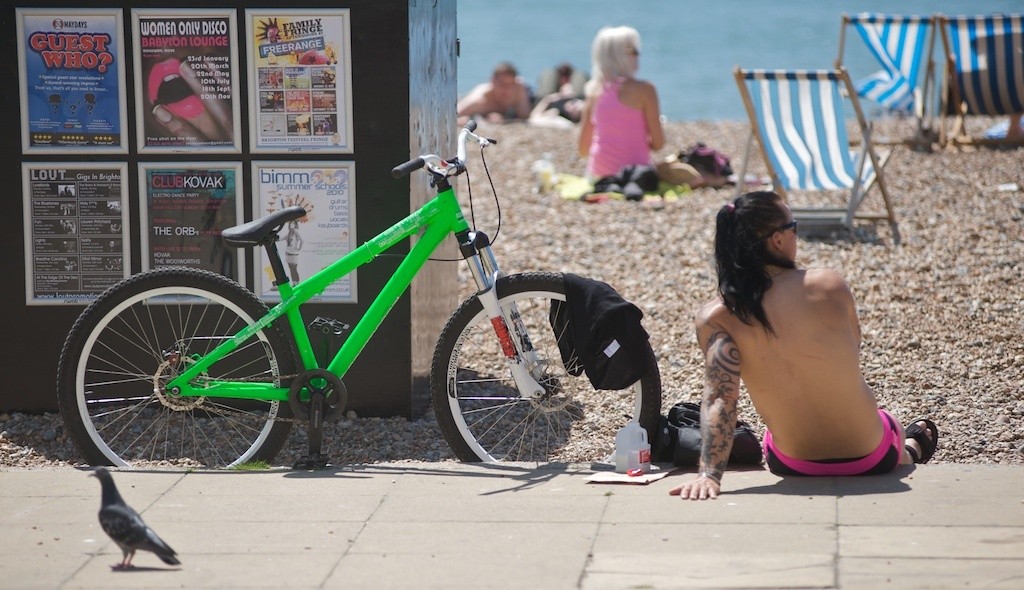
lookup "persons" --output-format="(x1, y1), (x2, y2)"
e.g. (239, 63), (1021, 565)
(667, 190), (939, 501)
(575, 26), (665, 182)
(454, 62), (588, 130)
(151, 56), (234, 147)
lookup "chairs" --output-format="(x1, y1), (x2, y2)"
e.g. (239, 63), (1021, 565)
(833, 15), (1024, 145)
(729, 64), (894, 235)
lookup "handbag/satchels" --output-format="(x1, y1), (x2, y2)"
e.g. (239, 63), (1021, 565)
(664, 400), (763, 466)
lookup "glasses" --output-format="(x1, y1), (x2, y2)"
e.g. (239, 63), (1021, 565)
(761, 220), (799, 239)
(623, 47), (639, 56)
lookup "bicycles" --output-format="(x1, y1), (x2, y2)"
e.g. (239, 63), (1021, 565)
(52, 118), (666, 477)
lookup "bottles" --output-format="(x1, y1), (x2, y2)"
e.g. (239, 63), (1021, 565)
(616, 422), (650, 473)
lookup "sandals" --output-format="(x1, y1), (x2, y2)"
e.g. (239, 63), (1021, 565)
(905, 418), (937, 463)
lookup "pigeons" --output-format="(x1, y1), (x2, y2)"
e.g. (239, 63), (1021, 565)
(88, 466), (180, 569)
